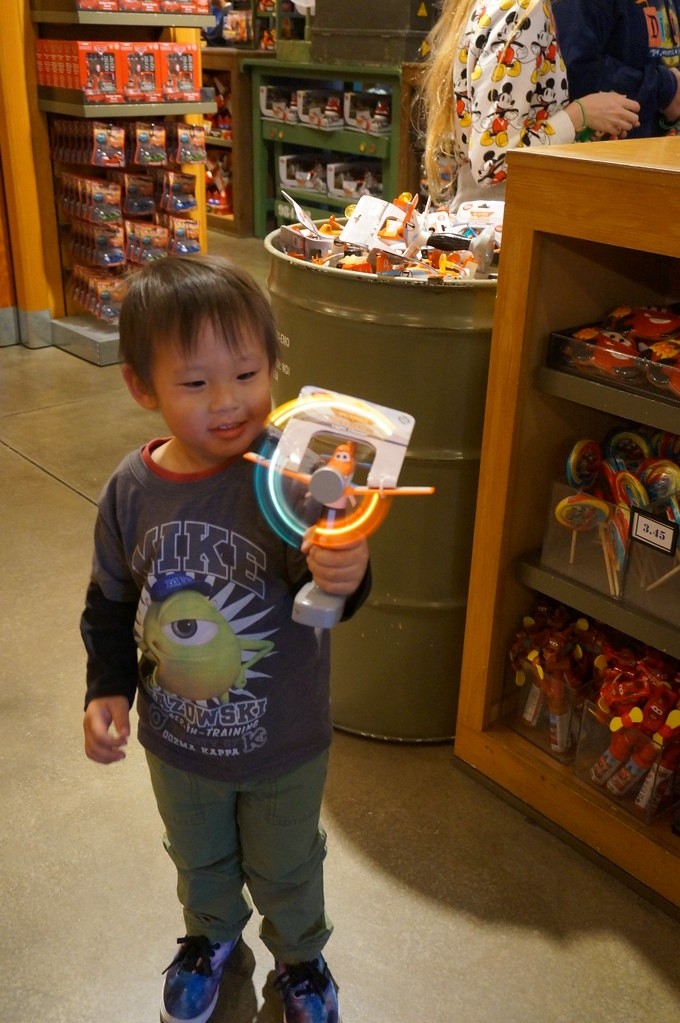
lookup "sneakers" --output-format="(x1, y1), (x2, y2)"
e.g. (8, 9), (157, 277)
(272, 952), (339, 1023)
(160, 934), (240, 1023)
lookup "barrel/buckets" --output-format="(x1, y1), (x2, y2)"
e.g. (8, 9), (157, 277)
(264, 218), (496, 745)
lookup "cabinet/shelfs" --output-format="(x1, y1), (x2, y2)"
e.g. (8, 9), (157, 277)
(399, 60), (430, 212)
(0, 11), (218, 367)
(201, 47), (275, 238)
(201, 0), (311, 50)
(455, 133), (680, 904)
(247, 57), (402, 241)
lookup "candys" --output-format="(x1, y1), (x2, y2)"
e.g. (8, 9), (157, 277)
(553, 428), (679, 597)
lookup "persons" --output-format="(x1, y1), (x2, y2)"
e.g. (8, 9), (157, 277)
(552, 0), (680, 139)
(414, 1), (641, 215)
(77, 250), (375, 1022)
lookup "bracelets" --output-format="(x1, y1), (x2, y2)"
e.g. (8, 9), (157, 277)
(575, 100), (587, 129)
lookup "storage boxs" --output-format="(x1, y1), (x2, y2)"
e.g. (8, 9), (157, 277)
(624, 508), (680, 629)
(542, 330), (680, 408)
(572, 700), (680, 825)
(537, 463), (631, 601)
(31, 0), (210, 15)
(500, 652), (583, 767)
(34, 37), (200, 106)
